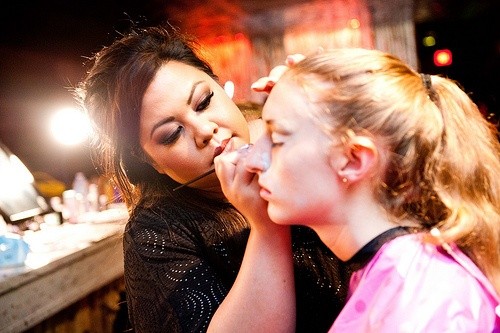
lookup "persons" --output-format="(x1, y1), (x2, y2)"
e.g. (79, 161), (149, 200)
(247, 45), (499, 332)
(66, 15), (345, 333)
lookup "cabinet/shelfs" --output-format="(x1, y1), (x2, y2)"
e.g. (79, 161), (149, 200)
(0, 224), (125, 333)
